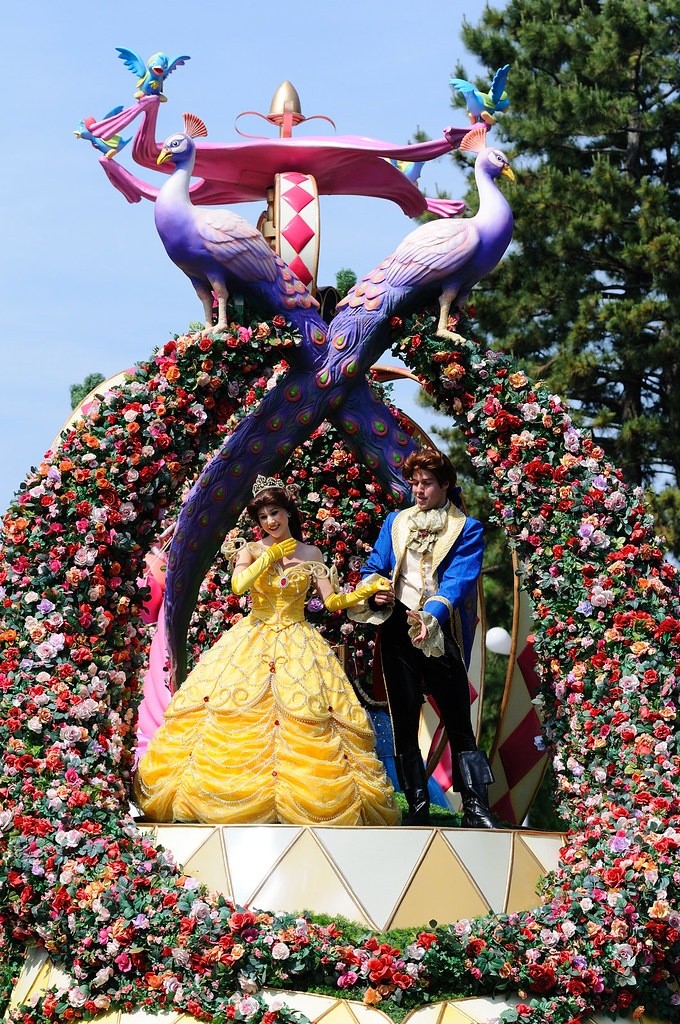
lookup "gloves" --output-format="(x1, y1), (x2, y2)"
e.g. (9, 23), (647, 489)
(232, 538), (297, 595)
(324, 578), (390, 612)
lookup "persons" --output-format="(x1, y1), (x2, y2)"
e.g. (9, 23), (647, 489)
(133, 474), (403, 824)
(347, 449), (507, 829)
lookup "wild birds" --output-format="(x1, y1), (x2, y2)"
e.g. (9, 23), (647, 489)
(71, 47), (515, 813)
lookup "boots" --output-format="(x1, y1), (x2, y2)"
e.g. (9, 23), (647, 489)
(452, 750), (513, 829)
(395, 750), (430, 826)
